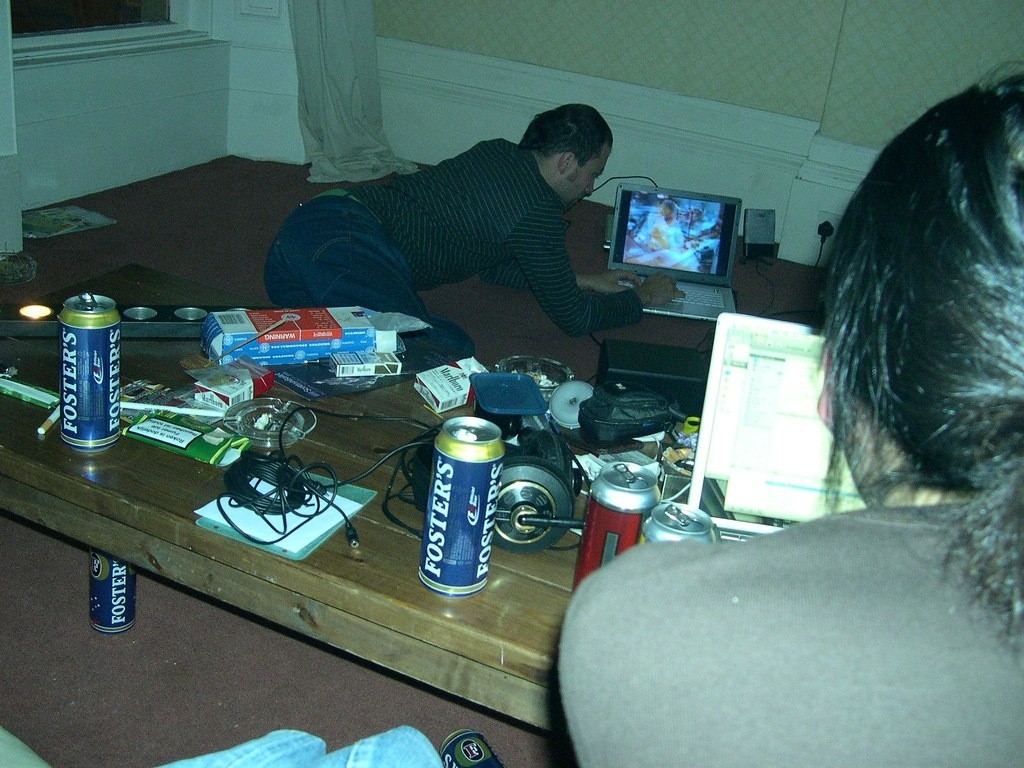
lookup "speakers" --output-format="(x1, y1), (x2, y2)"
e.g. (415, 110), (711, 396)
(743, 209), (775, 259)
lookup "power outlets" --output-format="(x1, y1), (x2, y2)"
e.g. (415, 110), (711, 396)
(815, 210), (845, 243)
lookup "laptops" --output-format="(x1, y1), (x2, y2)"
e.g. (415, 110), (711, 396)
(609, 182), (742, 321)
(686, 313), (866, 542)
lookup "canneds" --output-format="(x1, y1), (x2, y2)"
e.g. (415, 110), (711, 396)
(418, 416), (504, 598)
(439, 729), (507, 768)
(89, 544), (138, 634)
(638, 503), (714, 545)
(57, 291), (122, 453)
(571, 462), (661, 593)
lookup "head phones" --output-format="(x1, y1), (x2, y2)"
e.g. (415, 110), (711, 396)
(411, 426), (582, 554)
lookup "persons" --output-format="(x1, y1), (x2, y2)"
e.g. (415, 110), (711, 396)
(262, 103), (686, 358)
(557, 59), (1024, 766)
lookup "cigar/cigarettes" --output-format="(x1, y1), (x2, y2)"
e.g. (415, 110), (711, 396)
(36, 404), (60, 434)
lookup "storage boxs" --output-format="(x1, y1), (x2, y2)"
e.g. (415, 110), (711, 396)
(411, 354), (489, 413)
(200, 304), (407, 366)
(329, 352), (403, 378)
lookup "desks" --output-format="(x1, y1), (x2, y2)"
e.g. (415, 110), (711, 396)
(0, 261), (698, 741)
